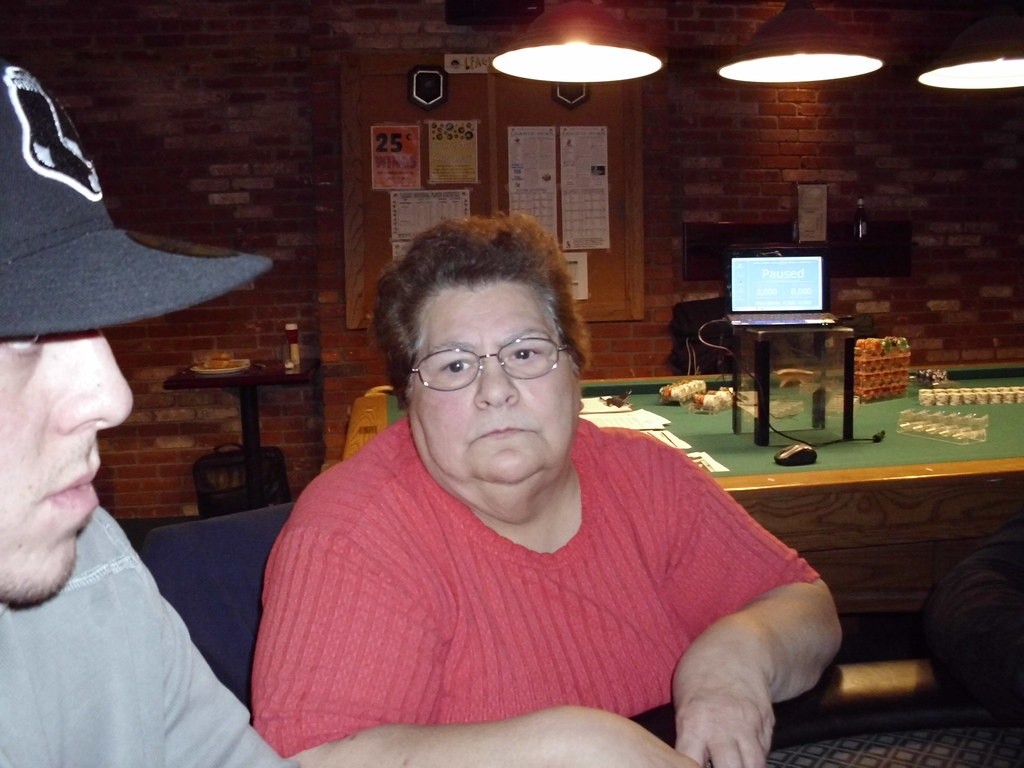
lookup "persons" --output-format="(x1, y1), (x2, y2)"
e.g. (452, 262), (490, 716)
(0, 61), (291, 768)
(251, 211), (842, 768)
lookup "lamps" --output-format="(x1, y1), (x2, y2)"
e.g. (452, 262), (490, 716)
(916, 0), (1024, 93)
(491, 0), (664, 84)
(714, 0), (886, 85)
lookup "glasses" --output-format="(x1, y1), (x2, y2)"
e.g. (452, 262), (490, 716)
(409, 337), (570, 392)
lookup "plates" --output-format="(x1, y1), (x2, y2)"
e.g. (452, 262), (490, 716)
(190, 358), (250, 374)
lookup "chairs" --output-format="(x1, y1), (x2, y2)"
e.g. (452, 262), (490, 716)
(140, 502), (295, 711)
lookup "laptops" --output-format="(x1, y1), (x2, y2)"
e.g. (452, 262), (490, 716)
(722, 250), (835, 330)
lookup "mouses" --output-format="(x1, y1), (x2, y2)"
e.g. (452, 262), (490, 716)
(774, 444), (818, 467)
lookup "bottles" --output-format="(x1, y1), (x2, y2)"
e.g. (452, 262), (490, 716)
(285, 324), (300, 365)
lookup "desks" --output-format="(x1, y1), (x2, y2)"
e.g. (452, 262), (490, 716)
(629, 702), (1024, 768)
(161, 358), (321, 499)
(343, 358), (1024, 615)
(732, 325), (856, 447)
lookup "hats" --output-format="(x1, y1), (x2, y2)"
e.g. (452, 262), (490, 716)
(0, 55), (273, 340)
